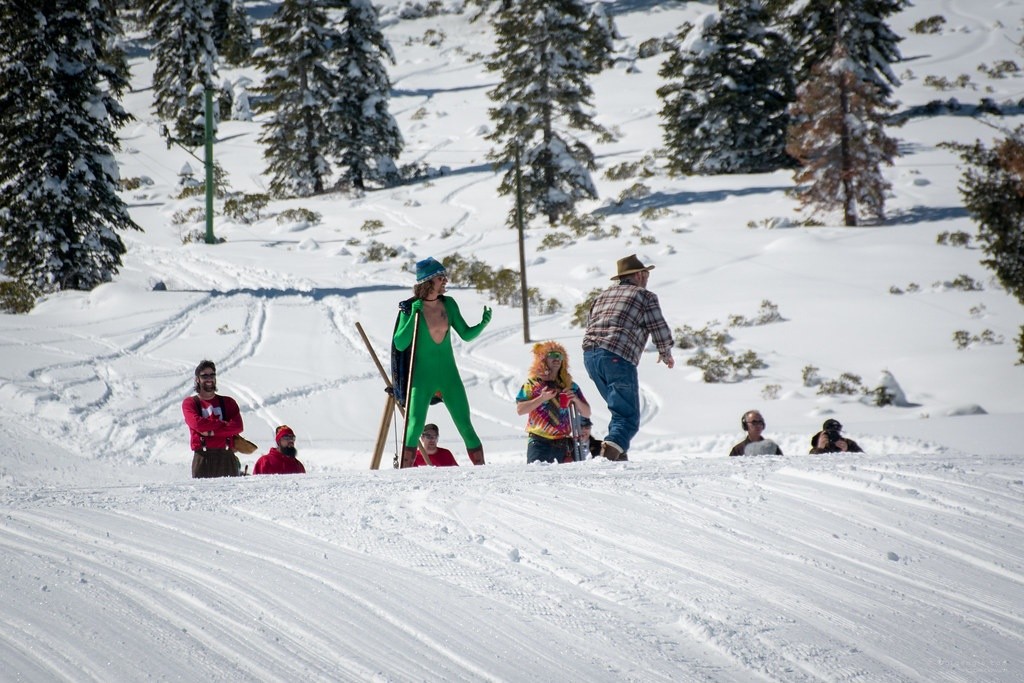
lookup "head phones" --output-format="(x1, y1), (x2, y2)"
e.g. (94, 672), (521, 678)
(742, 412), (767, 432)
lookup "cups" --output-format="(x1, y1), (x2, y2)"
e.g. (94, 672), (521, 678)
(559, 393), (570, 408)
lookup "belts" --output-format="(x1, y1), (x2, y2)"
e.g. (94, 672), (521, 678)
(584, 346), (599, 351)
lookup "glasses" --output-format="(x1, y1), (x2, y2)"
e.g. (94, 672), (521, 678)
(438, 276), (445, 281)
(747, 420), (763, 425)
(282, 435), (296, 442)
(425, 434), (437, 439)
(199, 373), (216, 380)
(547, 353), (563, 361)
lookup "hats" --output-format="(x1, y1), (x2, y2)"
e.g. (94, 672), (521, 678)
(610, 254), (655, 280)
(573, 416), (593, 427)
(275, 425), (294, 445)
(415, 257), (446, 284)
(823, 419), (842, 431)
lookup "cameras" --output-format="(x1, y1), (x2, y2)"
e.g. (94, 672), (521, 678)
(826, 431), (840, 444)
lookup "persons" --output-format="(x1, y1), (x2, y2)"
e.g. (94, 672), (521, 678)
(413, 423), (459, 467)
(183, 360), (244, 478)
(574, 415), (604, 460)
(809, 419), (864, 454)
(730, 410), (783, 458)
(391, 258), (492, 464)
(582, 254), (676, 461)
(253, 425), (306, 476)
(516, 340), (592, 464)
